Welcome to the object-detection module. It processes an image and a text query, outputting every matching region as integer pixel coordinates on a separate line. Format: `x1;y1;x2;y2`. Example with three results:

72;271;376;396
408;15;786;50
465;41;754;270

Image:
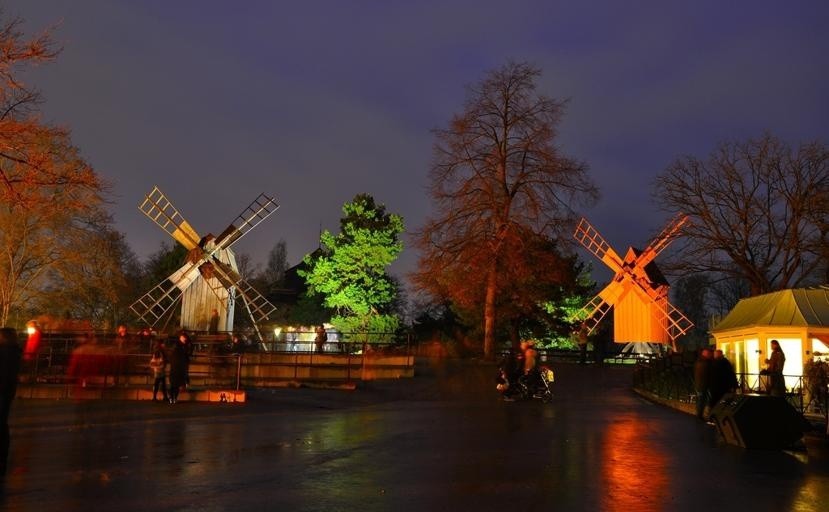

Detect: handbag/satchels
497;375;509;392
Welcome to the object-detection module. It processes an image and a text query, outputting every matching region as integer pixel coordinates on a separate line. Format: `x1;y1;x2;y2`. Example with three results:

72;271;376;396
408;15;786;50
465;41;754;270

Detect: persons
764;339;787;398
208;309;220;334
714;349;739;399
169;330;191;404
494;348;516;402
314;323;329;352
147;339;169;402
519;340;541;399
21;317;151;404
696;349;713;421
0;325;18;481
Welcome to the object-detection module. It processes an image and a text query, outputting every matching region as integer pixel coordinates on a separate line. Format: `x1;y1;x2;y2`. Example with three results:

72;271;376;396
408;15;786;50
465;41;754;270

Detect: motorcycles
492;357;557;406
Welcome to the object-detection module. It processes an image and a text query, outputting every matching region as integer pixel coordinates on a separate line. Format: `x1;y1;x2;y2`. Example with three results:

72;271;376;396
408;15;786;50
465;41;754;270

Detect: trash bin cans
708;393;816;449
